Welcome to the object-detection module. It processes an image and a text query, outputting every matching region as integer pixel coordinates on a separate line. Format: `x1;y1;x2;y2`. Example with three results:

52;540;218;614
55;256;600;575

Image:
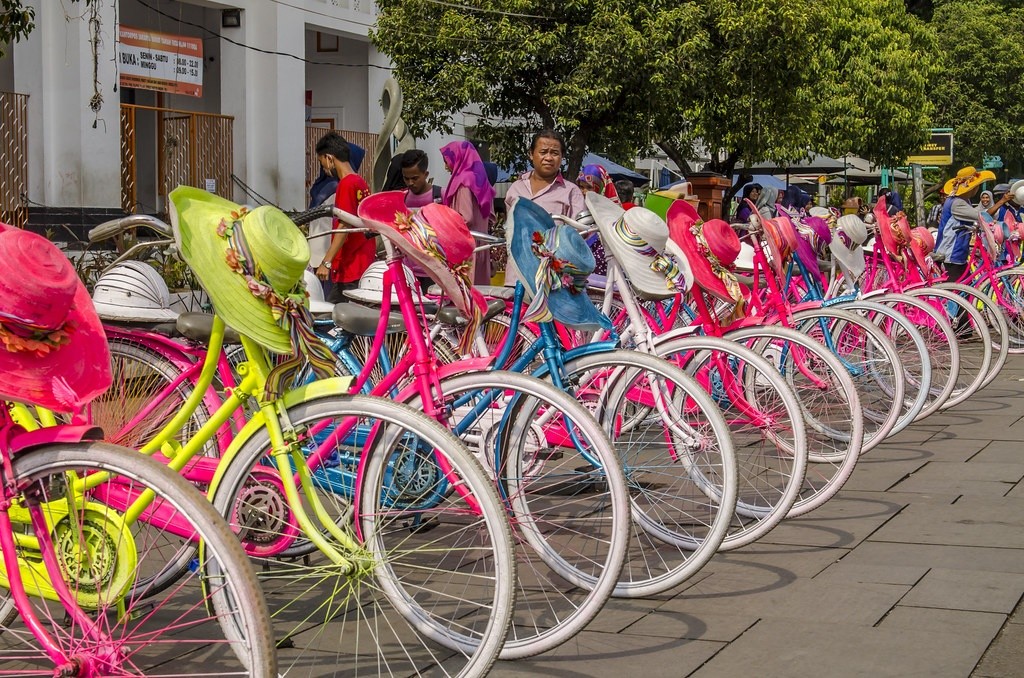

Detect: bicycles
0;191;1023;677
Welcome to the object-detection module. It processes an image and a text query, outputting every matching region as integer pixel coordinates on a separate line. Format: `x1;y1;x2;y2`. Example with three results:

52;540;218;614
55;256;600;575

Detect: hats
0;223;114;413
586;190;694;298
1009;179;1024;206
908;227;934;276
746;199;798;286
808;206;867;277
168;184;316;355
665;199;752;302
873;195;912;262
357;190;488;320
774;203;831;280
504;196;611;330
944;166;996;196
1002;209;1024;268
979;211;1003;263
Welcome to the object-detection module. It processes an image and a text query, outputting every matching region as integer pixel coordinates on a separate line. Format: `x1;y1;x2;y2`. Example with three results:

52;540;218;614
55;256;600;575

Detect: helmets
304;269;335;313
91;258;180;322
342;262;438;304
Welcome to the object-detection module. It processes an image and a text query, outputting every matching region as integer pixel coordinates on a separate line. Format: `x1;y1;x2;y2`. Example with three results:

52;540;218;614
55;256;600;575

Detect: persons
926;166;1024;342
307;128;640;297
735;184;903;276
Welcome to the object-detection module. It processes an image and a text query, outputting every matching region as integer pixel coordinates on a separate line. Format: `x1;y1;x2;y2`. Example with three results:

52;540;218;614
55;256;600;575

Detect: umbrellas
490;150;651;188
658;148;937;197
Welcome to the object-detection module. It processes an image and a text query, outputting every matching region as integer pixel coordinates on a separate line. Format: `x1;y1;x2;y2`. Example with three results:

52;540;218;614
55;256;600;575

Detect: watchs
322;261;332;269
